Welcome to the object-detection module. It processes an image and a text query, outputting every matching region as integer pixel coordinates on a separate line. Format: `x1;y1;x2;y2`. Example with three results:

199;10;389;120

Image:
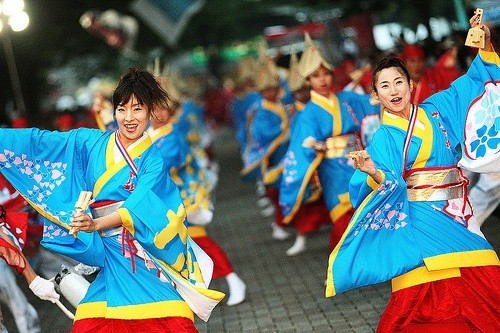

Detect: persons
0;5;500;333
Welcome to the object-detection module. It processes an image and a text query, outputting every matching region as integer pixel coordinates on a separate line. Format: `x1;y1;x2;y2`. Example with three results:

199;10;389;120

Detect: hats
154;58;184;108
288;48;310;92
299;30;336;77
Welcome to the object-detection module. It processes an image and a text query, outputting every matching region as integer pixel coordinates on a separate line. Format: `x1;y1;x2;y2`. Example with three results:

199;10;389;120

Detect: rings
356;163;361;166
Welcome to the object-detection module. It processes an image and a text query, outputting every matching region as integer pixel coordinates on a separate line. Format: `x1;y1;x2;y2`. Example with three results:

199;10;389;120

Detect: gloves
28;275;61;304
